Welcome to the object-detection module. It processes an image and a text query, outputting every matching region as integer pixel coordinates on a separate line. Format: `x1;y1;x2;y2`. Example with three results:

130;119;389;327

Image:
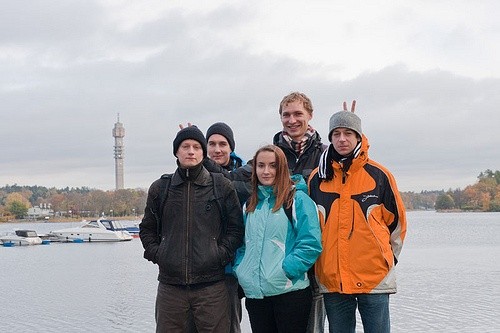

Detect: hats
328;112;363;142
206;122;235;152
173;125;207;159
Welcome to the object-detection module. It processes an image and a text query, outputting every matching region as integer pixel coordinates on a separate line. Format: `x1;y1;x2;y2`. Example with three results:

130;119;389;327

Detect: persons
206;122;246;333
139;127;246;333
308;111;407;333
224;144;323;333
178;92;356;333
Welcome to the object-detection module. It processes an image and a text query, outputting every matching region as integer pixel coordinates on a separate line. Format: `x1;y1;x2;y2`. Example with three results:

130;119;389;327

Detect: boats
0;230;49;246
50;216;132;242
124;227;140;238
